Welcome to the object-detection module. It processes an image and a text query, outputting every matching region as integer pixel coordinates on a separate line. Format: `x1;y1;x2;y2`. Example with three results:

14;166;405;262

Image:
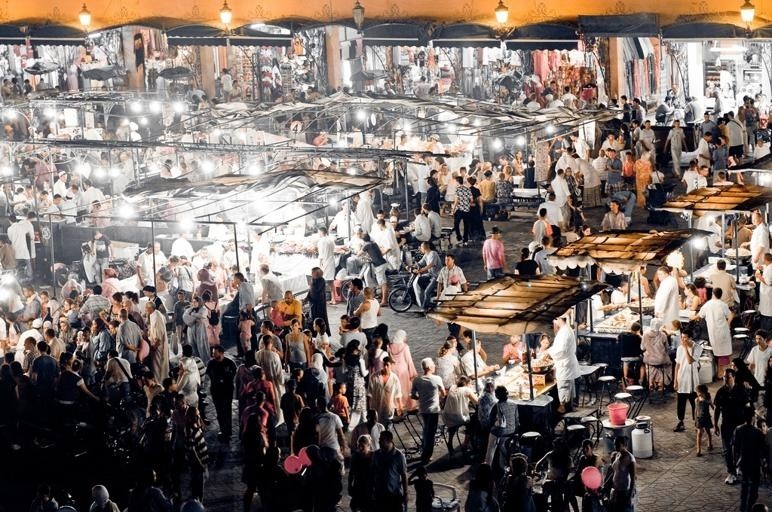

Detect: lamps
495;0;509;42
740;0;755;38
218;0;233;32
79;3;91;32
351;0;365;34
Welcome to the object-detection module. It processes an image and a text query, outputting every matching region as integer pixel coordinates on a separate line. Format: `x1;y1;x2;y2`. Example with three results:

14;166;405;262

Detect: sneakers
673;421;743;485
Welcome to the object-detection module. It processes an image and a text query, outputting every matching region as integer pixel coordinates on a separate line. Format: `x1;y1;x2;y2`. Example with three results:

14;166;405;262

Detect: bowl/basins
519;362;555;373
724;253;752;261
626;302;654;314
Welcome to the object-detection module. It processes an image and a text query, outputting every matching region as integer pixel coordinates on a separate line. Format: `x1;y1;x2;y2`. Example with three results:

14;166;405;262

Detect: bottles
756;269;761;283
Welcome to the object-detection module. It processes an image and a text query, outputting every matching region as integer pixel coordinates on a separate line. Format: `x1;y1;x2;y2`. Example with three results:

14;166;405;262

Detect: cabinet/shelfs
702;59;736;99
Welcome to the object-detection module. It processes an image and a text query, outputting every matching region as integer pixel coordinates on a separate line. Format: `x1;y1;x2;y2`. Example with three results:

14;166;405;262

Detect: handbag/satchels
129;378;141;394
493;411;508;429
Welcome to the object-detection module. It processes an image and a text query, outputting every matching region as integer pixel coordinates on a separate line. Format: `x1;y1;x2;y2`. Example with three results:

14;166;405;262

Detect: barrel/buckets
631;422;654;459
606;402;630;425
699;355;714;384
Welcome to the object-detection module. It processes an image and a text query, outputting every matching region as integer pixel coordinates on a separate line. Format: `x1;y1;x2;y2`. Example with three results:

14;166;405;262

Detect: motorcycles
389;263;444;314
340;251;398;305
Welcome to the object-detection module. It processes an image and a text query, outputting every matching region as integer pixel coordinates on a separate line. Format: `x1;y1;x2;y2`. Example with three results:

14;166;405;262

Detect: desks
510;174;548;210
641;106;720;162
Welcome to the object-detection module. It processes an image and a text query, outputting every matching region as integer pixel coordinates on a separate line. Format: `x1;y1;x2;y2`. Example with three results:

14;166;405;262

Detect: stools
732;309;757;346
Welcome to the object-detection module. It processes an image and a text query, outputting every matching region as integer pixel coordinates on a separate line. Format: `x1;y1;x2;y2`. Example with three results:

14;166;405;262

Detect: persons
0;41;771;511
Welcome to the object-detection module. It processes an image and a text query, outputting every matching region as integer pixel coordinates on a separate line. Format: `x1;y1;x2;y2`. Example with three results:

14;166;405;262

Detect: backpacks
203;302;220;326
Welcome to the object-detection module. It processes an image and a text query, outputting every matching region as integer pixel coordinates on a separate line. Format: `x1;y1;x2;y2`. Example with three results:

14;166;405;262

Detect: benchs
418;232;448;243
441;226;456;248
